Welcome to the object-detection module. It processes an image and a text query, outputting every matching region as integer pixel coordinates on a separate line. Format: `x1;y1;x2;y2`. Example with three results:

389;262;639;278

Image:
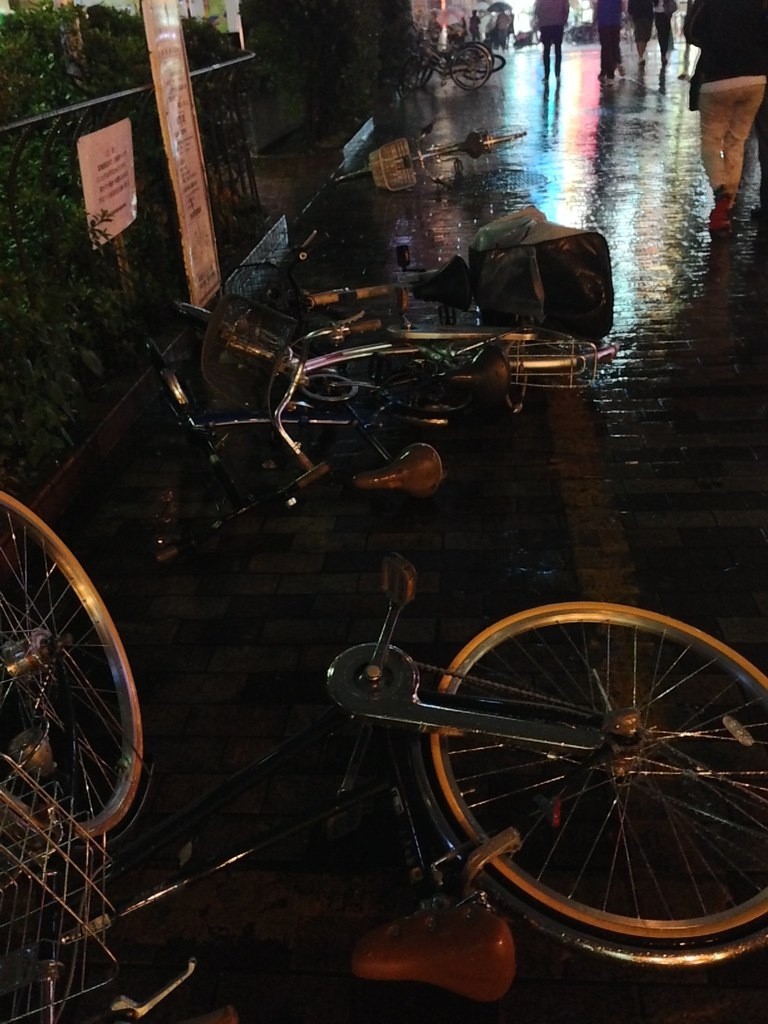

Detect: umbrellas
487;2;511;12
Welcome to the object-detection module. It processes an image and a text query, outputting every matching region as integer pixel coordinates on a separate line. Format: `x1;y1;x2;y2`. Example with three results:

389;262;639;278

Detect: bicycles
333;118;525;191
140;207;622;565
0;486;766;1024
393;14;507;98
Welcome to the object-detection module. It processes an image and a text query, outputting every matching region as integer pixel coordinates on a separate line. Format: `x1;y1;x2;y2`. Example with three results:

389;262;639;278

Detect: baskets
0;749;119;1024
200;292;299;417
223;262;288;312
369;138;417;192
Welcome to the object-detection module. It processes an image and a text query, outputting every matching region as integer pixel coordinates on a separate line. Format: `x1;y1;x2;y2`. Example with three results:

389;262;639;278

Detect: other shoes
607;79;617;85
598;75;606;84
618;64;625;76
751;206;768;219
661;56;668;65
678;74;686;79
638;57;646;66
710;193;731;229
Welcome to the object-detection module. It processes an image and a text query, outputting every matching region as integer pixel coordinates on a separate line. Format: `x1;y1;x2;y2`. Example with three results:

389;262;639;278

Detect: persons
208;16;220;33
417;9;511;53
535;0;570;80
683;0;768;232
591;0;677;86
